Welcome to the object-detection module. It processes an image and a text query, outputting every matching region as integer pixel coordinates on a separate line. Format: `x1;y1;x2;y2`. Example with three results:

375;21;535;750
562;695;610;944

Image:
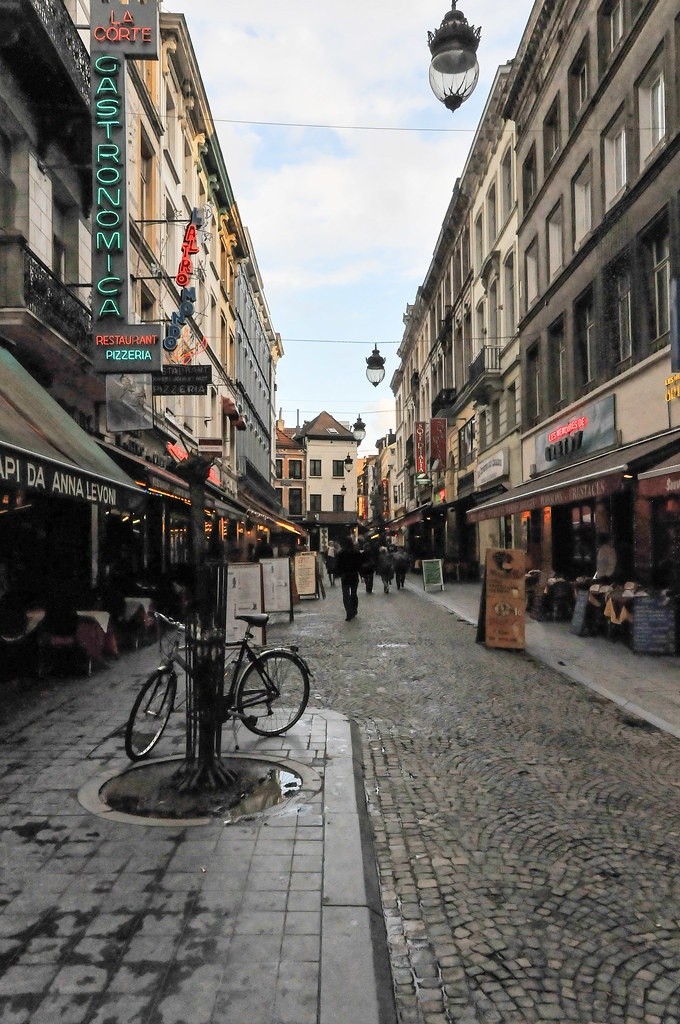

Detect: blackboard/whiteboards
151;364;213;395
422;558;445;592
632;596;678;658
569;590;591;636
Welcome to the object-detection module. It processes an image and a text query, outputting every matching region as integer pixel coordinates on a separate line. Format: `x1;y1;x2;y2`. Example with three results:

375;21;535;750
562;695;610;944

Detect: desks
0;580;165;687
524;569;680;647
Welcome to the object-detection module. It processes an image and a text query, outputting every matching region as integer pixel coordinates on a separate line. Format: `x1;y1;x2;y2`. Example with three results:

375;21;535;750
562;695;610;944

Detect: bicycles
123;610;316;761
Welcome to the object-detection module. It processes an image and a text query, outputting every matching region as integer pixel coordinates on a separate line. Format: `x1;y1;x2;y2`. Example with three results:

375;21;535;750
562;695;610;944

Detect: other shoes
346;608;359;620
383;587;390;593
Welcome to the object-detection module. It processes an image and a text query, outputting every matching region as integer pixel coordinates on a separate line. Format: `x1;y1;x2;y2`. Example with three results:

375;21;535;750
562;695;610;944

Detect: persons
357;547;369;583
252;534;274;566
392;546;411;590
377;544;394;594
268;536;338;587
592;530;620;582
333;536;366;624
246;541;256;563
359;541;378;595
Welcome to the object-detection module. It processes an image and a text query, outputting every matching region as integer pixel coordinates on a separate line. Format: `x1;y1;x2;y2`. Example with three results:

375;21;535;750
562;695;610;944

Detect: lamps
343;452;353;473
426;0;483;113
340;483;347;497
365;341;388;389
352;413;367;446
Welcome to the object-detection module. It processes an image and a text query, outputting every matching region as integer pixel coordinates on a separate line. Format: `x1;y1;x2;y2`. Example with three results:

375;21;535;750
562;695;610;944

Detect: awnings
86;431;214;512
242;506;303;538
465;433;680;523
0;328;145;516
636;444;680;500
189;480;249;525
